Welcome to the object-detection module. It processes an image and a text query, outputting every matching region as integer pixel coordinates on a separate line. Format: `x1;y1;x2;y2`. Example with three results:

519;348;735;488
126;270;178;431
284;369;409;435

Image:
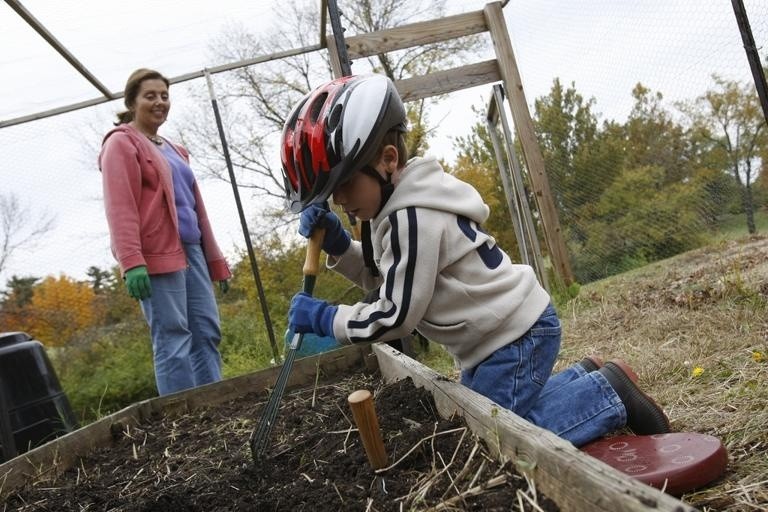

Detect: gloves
287;291;339;342
297;206;350;255
219;277;230;293
124;265;153;301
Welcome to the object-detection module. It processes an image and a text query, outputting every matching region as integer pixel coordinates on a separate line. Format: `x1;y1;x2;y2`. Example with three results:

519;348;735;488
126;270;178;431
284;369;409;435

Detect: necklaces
146;137;163;145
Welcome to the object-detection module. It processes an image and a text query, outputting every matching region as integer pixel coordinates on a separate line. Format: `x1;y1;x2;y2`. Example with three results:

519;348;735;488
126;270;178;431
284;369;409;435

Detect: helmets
279;72;407;215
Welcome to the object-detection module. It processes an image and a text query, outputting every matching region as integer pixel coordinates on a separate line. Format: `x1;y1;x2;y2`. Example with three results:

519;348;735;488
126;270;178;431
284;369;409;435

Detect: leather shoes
581;355;672;437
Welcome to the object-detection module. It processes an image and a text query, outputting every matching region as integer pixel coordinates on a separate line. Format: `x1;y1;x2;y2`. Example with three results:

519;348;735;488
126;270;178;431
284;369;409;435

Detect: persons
98;68;232;397
277;74;670;448
